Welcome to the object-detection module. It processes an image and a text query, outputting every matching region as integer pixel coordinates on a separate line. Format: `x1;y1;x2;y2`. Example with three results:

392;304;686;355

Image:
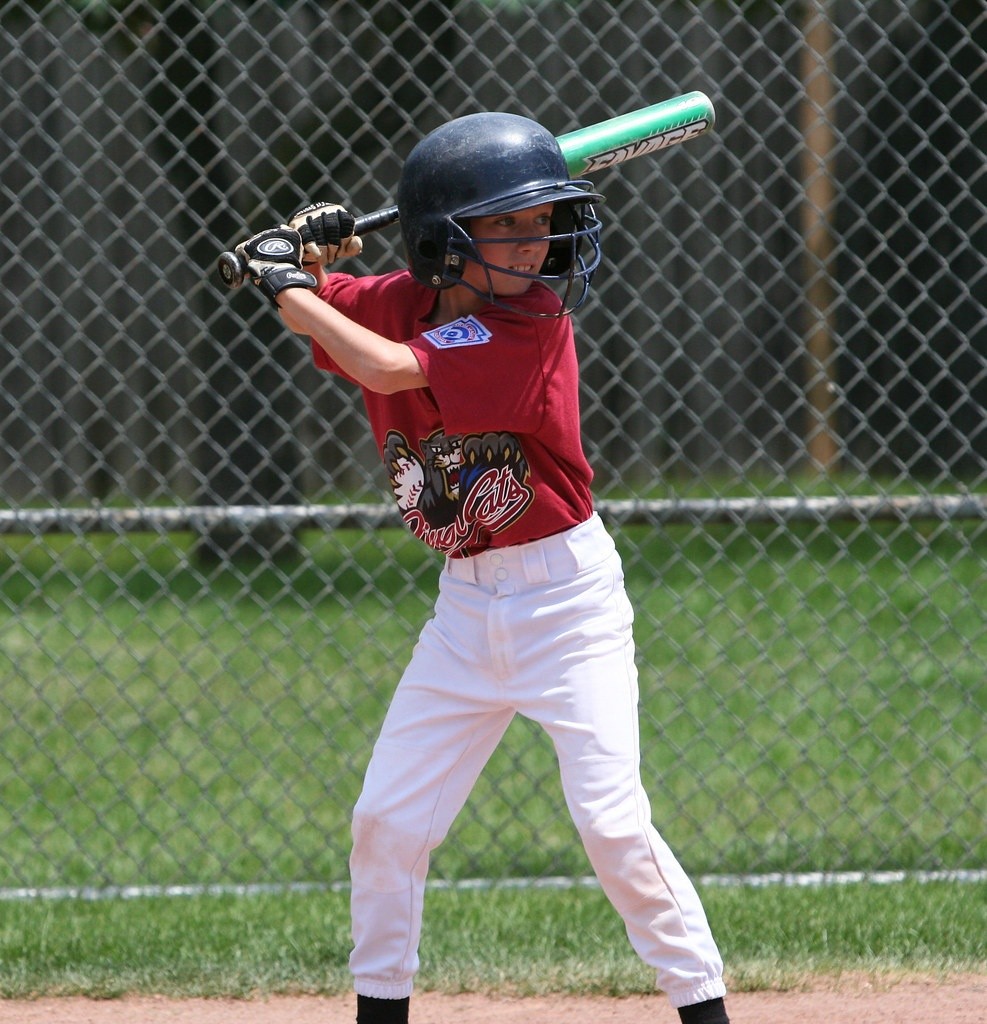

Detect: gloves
235;224;317;312
287;201;363;267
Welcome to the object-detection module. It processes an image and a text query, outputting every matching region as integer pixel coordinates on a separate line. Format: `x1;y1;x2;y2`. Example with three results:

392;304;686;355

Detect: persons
235;111;731;1024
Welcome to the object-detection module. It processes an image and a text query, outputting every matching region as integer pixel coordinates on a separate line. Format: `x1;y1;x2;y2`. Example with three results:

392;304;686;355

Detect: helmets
397;112;606;320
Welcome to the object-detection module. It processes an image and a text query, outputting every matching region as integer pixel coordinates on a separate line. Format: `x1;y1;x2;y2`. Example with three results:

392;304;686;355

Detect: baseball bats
219;90;718;289
